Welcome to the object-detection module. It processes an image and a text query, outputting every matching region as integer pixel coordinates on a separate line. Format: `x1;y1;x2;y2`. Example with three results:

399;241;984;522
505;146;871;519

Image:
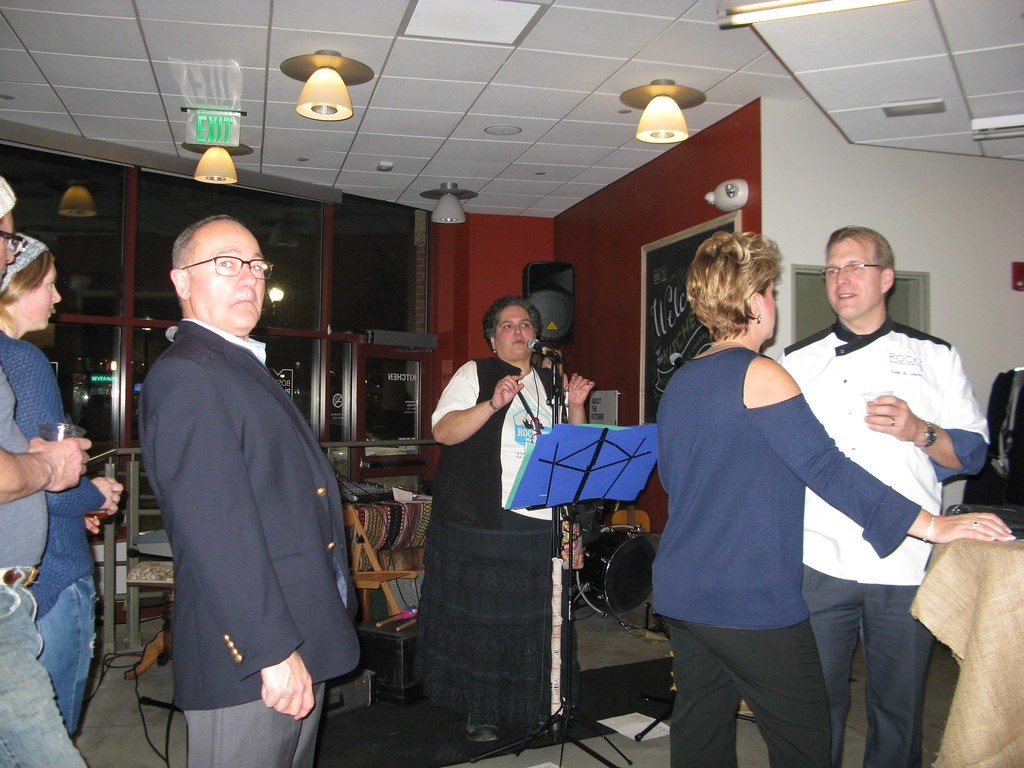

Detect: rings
890;417;895;427
499;379;503;383
972;521;980;529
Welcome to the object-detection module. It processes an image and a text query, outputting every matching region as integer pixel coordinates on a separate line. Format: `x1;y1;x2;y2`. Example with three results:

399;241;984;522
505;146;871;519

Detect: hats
0;175;17;220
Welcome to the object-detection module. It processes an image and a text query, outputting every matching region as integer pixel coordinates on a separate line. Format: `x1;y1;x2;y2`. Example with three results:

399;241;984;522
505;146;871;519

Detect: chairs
344;509;417;622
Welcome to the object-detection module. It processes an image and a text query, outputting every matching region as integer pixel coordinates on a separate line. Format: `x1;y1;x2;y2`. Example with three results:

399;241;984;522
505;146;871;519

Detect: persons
651;234;1016;768
0;173;123;768
418;298;595;742
138;214;362;768
770;225;991;768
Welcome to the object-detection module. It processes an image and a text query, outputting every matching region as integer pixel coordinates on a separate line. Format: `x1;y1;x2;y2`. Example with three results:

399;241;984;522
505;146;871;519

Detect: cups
38;422;86;442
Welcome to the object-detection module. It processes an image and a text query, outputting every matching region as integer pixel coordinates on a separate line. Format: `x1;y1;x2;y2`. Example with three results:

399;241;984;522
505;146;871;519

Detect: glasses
820;264;879;280
0;229;26;255
183;255;275;280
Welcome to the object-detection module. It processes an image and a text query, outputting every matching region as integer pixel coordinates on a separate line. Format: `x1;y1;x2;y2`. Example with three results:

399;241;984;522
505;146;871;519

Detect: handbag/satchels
560;506;584;569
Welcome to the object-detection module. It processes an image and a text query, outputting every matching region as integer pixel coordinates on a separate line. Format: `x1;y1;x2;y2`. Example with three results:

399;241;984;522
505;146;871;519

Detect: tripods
468;364;634;768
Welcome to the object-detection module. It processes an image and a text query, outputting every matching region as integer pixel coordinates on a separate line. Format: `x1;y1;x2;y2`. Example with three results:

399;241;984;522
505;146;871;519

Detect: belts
1;567;39;587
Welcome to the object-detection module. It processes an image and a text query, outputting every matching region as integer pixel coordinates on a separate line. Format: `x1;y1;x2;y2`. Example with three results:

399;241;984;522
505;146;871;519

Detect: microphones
527;338;563;364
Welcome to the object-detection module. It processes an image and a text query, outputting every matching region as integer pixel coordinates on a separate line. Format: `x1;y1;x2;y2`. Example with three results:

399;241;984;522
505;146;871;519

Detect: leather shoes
466;709;549;741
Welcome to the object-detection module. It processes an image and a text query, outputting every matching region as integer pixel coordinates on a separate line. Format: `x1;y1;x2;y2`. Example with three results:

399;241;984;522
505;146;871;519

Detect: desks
342;491;442;621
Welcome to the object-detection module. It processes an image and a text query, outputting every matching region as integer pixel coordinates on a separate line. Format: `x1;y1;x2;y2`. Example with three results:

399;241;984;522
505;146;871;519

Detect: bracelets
489;400;499;412
922;515;935;542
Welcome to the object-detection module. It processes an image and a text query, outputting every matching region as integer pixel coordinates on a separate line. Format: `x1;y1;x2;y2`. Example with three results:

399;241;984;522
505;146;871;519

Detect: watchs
914;418;937;448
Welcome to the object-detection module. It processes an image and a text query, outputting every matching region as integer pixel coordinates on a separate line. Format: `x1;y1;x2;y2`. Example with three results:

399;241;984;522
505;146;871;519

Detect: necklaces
710;341;754;351
529;364;543;419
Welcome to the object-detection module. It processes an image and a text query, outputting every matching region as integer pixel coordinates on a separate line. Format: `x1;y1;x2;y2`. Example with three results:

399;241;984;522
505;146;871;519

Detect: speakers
522;260;576;342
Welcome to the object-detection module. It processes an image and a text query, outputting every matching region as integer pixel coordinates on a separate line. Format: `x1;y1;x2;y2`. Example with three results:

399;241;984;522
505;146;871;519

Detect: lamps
419;183;479;223
621;77;707;145
716;0;913;31
275;51;377;122
179;141;251;187
970;112;1024;139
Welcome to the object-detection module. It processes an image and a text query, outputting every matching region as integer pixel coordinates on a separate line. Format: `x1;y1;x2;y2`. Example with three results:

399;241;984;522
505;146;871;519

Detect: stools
123;560;186;681
